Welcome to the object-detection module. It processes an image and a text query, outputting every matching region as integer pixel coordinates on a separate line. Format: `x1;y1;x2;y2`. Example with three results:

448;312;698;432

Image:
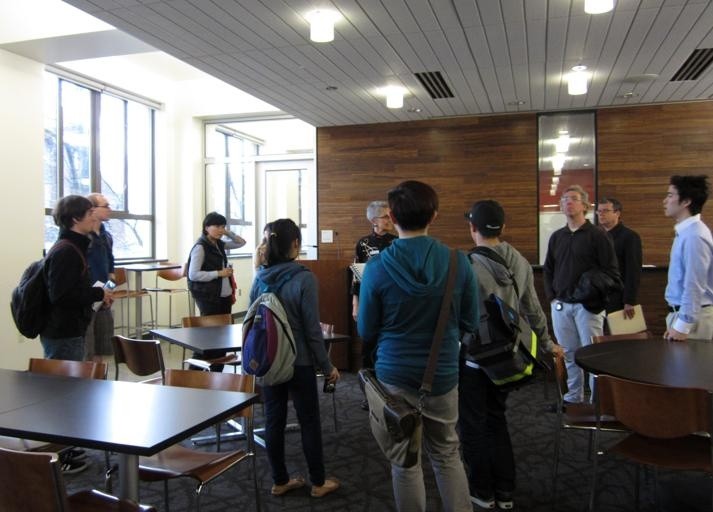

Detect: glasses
595;208;614;215
96;204;109;207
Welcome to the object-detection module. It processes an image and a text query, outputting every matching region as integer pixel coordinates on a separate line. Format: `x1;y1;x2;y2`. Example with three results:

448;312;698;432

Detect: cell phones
104;280;116;291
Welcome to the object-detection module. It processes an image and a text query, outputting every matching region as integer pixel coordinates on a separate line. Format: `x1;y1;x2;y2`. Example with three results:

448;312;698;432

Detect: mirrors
536;109;597;266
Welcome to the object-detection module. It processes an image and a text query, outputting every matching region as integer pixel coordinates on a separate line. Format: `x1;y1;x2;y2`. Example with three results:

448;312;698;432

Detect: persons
185;211;247;372
349;199;400;411
541;183;621;415
457;196;566;510
355;177;483;512
247;217;343;498
595;195;643;335
661;172;713;341
84;192;115;360
39;194;115;361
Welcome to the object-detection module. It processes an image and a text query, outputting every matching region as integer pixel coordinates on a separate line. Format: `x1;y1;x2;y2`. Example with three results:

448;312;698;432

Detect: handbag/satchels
467;292;552;392
357;368;422;468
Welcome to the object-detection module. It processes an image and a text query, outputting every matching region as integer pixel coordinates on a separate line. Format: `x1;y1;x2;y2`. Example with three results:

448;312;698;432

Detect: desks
150;322;346;447
114;263;181;334
0;366;256;507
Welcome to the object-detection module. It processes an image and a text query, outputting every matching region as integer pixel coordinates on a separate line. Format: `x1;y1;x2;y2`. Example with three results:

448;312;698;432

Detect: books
605;304;647;337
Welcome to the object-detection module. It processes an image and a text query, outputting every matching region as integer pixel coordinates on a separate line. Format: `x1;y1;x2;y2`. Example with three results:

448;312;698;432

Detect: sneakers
60;448;88;474
470;489;514;509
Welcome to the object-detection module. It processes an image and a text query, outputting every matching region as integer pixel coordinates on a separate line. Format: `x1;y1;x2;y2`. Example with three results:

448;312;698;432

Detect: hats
464;200;505;229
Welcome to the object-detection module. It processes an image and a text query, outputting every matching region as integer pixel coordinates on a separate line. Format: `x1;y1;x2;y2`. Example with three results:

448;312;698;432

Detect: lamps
584;0;613;14
568;78;587;95
549;131;568;196
386;90;403;109
309;15;334;42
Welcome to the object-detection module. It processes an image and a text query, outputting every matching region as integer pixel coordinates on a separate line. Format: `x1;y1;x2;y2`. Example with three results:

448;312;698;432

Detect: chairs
0;449;154;509
106;372;256;508
25;358;108;382
181;313;241;375
553;331;712;512
110;265;155;336
0;261;352;512
142;262;193;328
109;335;170;388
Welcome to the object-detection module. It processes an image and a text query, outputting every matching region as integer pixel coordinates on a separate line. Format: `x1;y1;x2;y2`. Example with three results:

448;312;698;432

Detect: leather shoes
271;478;304;495
311;478;340;497
545;400;582;413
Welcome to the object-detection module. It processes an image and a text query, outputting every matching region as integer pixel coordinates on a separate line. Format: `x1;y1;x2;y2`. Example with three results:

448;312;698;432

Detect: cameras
323;378;336;393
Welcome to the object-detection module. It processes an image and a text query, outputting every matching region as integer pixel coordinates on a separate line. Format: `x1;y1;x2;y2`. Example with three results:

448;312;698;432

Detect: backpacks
241;267;310;387
10;240;87;339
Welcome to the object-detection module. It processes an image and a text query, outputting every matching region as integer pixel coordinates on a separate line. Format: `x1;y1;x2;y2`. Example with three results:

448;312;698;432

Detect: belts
667;304;709;312
